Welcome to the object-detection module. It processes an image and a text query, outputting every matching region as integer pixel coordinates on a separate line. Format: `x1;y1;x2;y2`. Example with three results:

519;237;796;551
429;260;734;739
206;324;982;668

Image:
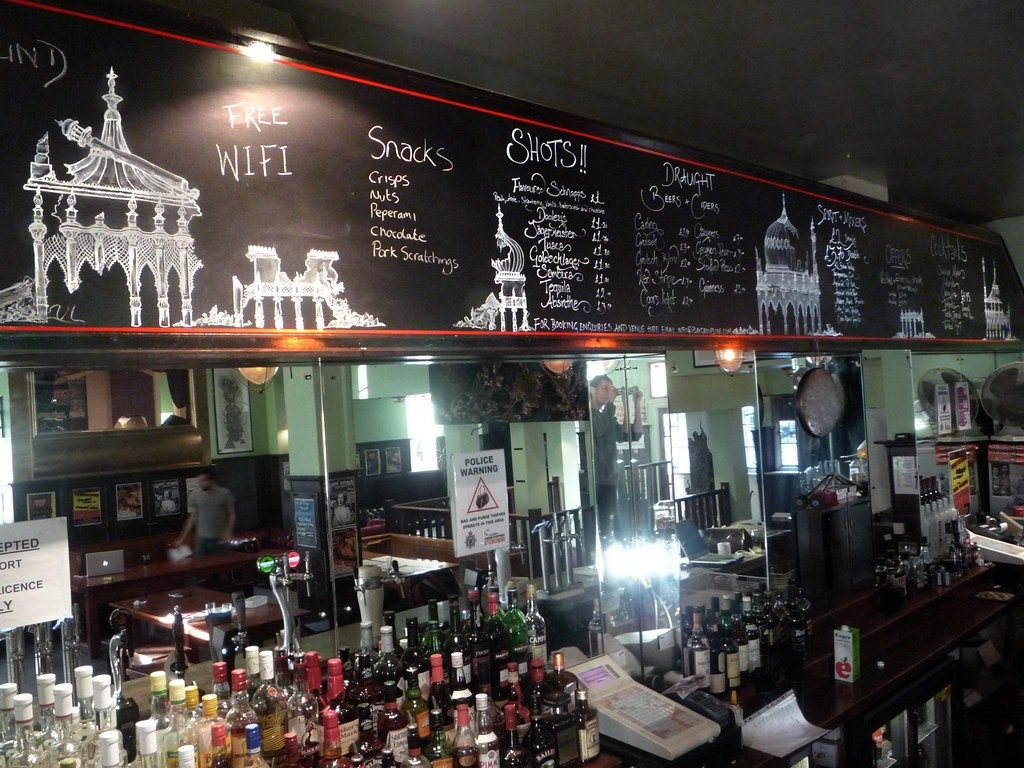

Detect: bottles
875;475;982;599
849;459;861;481
0;520;601;768
683;578;813;694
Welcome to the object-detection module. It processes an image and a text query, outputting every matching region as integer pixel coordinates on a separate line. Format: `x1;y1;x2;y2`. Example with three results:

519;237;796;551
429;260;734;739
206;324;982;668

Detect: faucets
282;553;292;603
303;550;314;597
391;560;405;598
120;614;135;662
231;591;247;659
352;566;367;607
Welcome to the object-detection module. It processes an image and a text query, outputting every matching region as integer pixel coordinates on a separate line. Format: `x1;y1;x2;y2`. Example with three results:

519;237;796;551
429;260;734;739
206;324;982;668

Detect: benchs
70;526;292;581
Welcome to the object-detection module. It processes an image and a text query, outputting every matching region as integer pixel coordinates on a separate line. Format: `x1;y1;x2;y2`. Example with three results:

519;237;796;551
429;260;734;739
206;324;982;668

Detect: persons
589;375;643;540
172;469;236;556
160;489;175;513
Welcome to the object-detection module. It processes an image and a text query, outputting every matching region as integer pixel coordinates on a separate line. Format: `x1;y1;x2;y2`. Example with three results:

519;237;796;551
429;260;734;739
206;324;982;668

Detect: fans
917;367;988;435
980;361;1024;432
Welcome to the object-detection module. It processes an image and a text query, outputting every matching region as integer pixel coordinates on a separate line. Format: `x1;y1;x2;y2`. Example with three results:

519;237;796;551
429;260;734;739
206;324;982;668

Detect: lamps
714;349;745;373
237;367;278;393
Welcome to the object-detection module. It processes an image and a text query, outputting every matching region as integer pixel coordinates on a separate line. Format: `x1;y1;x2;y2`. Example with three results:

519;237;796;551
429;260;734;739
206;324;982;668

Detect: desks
111;582;313;656
361;553;459;610
72;549;289;659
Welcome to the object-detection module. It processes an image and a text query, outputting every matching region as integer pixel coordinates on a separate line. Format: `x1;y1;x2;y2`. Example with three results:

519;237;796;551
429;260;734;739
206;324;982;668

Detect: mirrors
0;351;1024;701
31;369;197;444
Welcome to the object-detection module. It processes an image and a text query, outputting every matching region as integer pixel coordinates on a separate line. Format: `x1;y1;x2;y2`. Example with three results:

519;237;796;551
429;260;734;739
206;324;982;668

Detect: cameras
628;387;635;395
616;387;625;394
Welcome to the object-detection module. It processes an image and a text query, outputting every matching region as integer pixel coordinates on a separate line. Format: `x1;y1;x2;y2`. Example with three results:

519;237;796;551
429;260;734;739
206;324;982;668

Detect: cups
717;542;731;556
